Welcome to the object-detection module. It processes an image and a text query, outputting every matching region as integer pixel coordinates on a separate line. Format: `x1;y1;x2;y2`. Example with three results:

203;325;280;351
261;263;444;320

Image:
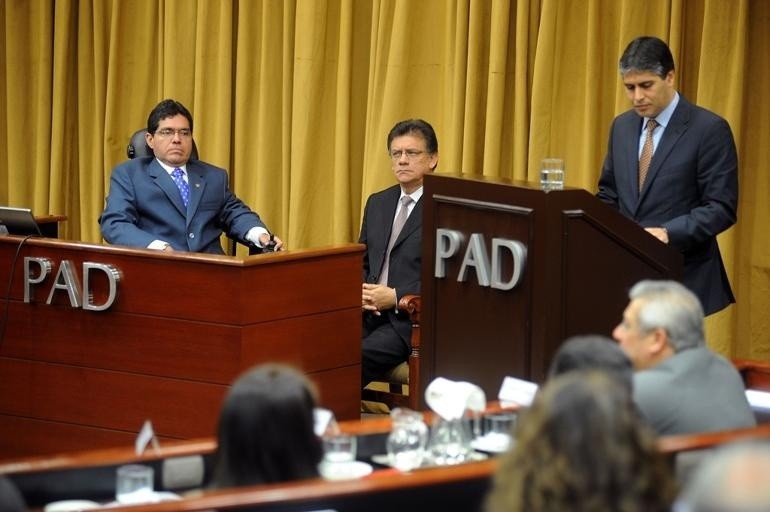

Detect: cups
485;411;517;451
541;158;565;190
386;407;427;469
323;434;356;465
116;465;153;505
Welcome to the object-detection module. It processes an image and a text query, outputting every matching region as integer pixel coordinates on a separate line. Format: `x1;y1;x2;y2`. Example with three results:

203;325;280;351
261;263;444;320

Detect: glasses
152;129;191;138
389;150;430;160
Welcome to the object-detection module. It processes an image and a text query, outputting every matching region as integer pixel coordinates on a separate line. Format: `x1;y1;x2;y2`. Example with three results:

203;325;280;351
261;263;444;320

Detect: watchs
162;243;171;251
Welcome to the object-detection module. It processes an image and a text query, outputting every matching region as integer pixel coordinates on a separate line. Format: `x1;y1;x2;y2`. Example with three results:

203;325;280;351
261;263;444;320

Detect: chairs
128;127;283;255
362;295;421;410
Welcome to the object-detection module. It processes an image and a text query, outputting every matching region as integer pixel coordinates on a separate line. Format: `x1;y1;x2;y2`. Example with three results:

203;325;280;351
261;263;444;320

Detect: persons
484;369;679;511
548;335;635;398
97;99;285;256
594;35;739;319
612;278;759;481
211;362;325;489
358;118;440;392
672;439;770;512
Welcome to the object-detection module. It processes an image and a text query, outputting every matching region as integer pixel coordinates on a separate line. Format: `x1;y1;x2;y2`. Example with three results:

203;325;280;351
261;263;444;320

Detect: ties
639;120;658;193
171;166;189;208
378;196;413;287
